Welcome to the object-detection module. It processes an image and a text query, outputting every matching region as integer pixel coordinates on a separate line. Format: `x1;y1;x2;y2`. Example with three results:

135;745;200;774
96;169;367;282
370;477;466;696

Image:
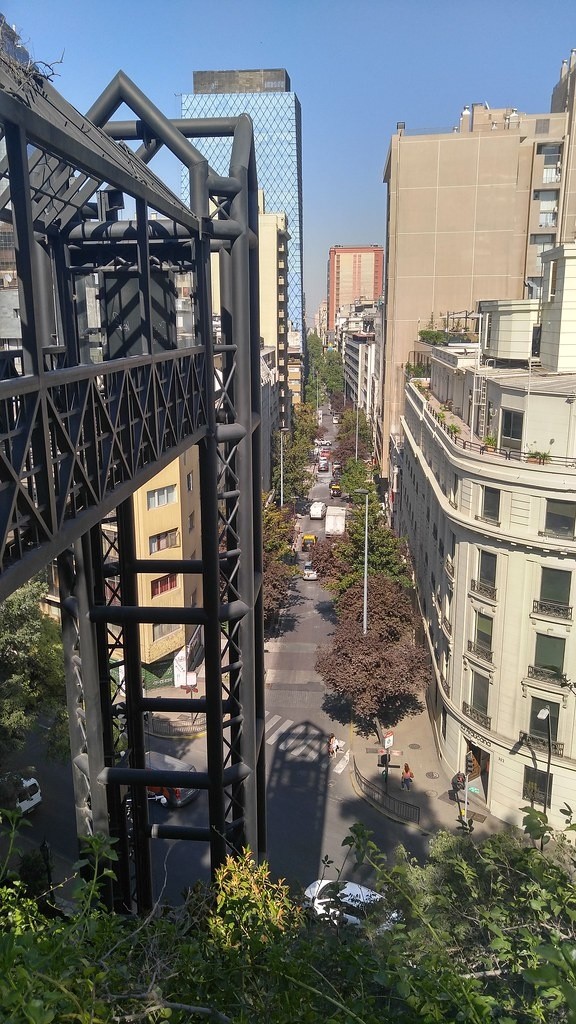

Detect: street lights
279;428;291;506
354;489;369;635
536;705;551;854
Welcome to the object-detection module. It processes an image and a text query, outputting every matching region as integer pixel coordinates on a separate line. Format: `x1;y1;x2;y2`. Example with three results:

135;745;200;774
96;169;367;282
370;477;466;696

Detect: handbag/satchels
409;769;414;778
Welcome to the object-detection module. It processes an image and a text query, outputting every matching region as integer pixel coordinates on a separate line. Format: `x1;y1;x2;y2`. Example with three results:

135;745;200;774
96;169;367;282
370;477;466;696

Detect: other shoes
406;790;410;792
400;788;404;791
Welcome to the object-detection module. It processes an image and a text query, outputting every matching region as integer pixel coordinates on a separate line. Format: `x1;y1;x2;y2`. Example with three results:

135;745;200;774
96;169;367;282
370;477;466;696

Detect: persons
401;763;414;791
328;733;338;758
452;773;466;802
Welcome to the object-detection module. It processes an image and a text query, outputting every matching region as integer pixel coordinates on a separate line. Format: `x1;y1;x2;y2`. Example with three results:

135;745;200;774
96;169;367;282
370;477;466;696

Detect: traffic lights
457;773;464;790
465;751;475;776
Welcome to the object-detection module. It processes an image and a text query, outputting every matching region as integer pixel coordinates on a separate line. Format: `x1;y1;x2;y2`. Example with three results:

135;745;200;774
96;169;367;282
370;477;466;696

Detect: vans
140;751;200;807
310;502;328;519
16;778;42;818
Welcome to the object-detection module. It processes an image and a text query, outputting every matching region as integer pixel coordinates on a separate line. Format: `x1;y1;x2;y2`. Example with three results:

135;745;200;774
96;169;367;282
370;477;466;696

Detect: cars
314;437;332;446
333;417;339;424
304;879;398;935
301;535;318;552
303;562;320;582
311;447;343;497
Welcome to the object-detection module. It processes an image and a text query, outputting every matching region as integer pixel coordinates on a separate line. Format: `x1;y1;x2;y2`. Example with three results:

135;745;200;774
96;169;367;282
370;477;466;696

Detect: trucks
324;506;349;538
